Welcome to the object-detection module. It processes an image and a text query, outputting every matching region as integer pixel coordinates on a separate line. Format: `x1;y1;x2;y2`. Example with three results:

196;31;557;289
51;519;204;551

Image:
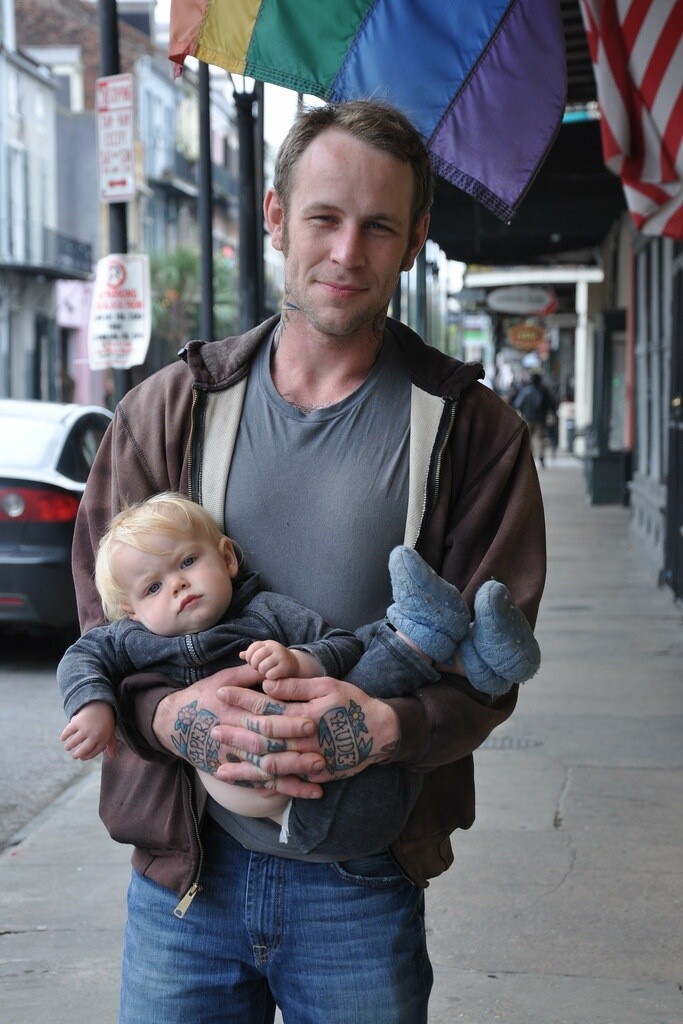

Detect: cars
0;395;116;628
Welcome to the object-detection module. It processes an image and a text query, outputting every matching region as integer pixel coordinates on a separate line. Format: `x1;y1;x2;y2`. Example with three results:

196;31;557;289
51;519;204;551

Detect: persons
56;494;540;858
512;373;557;467
71;101;546;1023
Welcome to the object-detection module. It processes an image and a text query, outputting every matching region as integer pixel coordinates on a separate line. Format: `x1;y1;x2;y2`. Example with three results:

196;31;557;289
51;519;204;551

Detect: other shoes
387;544;470;663
539;457;546;467
457;578;541;697
